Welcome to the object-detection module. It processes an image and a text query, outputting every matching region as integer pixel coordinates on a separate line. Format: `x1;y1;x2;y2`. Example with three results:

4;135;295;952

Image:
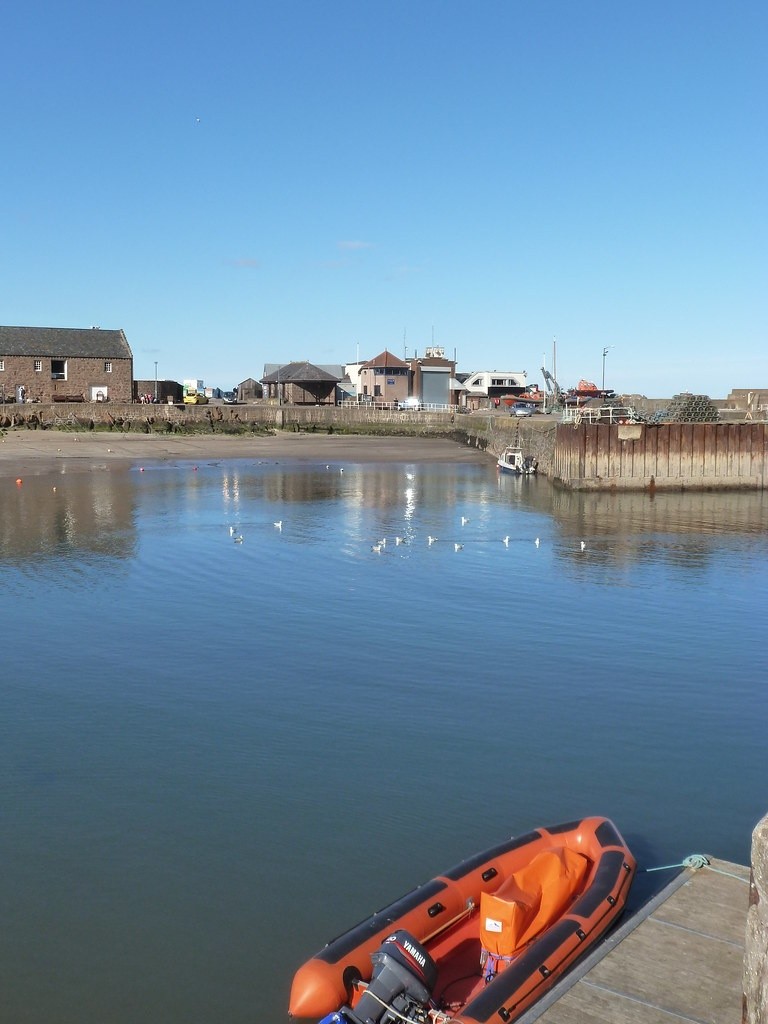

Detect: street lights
602;345;615;402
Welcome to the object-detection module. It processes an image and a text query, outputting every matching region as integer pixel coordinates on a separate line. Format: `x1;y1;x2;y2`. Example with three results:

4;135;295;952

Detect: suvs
398;396;423;411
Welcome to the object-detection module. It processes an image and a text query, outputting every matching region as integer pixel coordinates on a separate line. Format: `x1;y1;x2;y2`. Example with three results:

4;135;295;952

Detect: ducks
534;538;541;544
580;541;586;549
229;527;244;544
370;517;470;550
273;520;282;526
502;536;511;542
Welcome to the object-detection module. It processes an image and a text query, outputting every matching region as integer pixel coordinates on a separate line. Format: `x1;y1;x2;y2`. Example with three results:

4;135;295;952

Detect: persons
140;393;154;405
393;398;398;410
20;388;26;404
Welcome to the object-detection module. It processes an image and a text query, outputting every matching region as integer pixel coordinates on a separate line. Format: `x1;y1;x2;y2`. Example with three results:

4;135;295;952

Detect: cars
222;391;237;405
509;402;533;417
184;393;209;405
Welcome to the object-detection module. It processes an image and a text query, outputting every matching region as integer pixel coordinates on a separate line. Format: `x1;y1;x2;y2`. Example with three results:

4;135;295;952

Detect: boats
287;816;637;1024
496;445;536;473
